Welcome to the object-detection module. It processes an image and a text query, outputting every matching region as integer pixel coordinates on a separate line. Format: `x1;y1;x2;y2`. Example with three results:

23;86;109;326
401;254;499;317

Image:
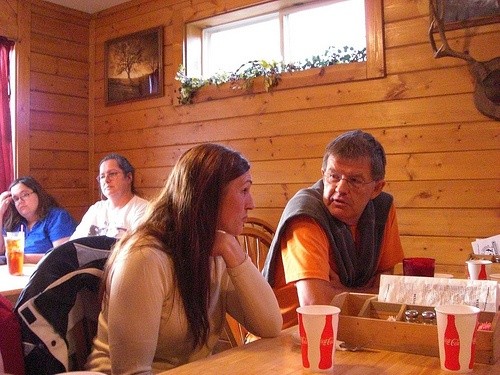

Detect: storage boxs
327;292;500;365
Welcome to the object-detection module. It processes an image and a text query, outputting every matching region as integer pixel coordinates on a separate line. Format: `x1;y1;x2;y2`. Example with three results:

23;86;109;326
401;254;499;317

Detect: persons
0;176;78;264
70;154;155;241
245;128;405;345
82;142;284;375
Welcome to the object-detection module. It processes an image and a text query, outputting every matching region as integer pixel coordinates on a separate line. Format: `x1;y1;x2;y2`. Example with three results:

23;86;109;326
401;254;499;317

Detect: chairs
13;218;278;375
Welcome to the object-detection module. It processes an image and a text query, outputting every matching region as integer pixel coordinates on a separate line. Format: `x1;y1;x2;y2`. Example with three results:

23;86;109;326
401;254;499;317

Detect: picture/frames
103;25;165;107
429;0;500;33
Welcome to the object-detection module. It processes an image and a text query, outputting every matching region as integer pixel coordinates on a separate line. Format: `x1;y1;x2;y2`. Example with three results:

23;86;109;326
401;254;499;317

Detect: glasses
324;170;372;191
96;171;123;182
13;191;33;201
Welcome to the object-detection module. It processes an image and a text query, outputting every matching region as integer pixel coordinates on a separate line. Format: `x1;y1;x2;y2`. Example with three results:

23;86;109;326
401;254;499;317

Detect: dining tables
0;262;39;304
153;323;500;375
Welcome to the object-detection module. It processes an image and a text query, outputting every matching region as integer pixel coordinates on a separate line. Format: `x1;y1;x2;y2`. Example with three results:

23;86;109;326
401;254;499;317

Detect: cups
433;305;481;374
490;273;500;282
434;274;455;279
4;236;26;274
8;231;25;239
466;259;493;280
402;257;436;277
296;304;341;370
